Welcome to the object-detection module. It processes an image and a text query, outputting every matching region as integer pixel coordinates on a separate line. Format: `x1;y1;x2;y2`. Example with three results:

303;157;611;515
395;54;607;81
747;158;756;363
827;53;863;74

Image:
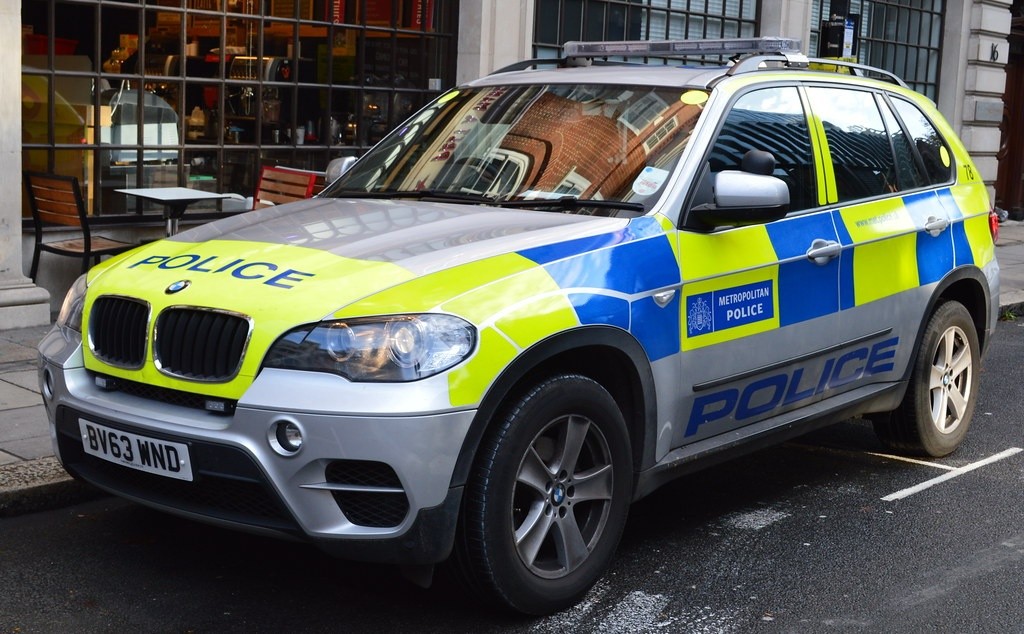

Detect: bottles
240;86;255;116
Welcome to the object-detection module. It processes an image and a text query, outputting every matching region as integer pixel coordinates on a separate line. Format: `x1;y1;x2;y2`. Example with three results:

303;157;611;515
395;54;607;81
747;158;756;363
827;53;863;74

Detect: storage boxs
155;0;435;33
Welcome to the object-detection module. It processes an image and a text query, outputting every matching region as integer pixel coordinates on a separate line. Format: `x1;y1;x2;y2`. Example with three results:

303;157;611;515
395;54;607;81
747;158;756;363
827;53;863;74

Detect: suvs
38;37;1001;618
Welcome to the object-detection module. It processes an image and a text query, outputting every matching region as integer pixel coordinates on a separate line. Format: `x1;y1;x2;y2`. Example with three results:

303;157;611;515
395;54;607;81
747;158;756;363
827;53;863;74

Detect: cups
271;129;281;145
296;127;304;144
232;131;244;144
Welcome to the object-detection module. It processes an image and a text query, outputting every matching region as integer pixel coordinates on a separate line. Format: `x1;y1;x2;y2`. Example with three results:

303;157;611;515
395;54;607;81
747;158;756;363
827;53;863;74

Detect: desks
112;187;232;238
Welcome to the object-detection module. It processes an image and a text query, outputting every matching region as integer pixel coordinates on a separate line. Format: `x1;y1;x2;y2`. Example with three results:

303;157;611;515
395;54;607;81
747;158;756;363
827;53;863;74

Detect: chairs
21;168;138;286
251;166;329;211
